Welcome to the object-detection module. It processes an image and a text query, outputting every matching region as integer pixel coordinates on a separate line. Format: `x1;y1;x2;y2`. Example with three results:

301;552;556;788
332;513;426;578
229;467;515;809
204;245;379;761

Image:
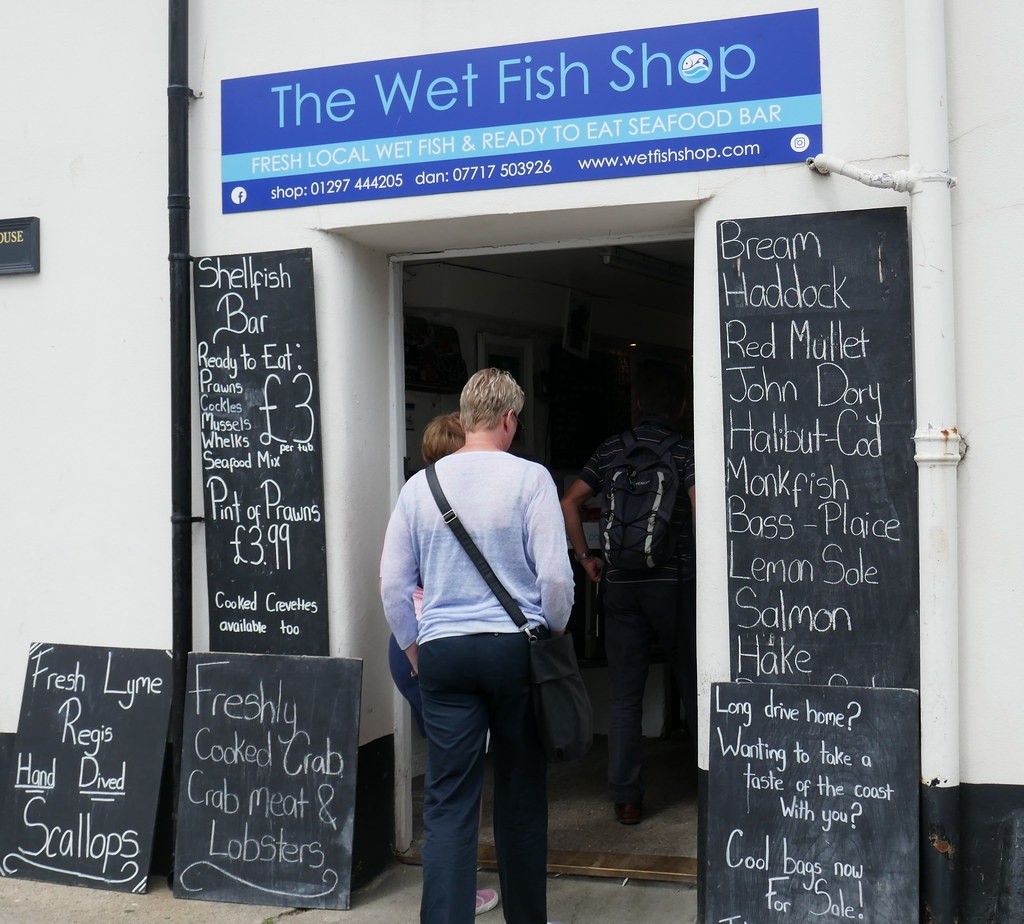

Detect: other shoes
475;889;498;916
615;803;640;825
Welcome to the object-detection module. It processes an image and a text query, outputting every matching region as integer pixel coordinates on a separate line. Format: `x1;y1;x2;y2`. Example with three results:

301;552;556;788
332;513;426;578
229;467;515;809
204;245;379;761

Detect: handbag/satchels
528;638;593;762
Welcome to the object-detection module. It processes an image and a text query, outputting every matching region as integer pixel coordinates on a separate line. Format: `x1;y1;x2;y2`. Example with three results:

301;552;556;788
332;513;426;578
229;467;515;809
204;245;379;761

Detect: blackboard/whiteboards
703;681;920;924
714;204;922;693
0;641;173;894
170;651;366;910
193;247;331;657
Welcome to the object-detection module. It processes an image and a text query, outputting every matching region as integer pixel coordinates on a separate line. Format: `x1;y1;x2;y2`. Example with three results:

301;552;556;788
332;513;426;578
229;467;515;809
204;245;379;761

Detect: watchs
574;550;593;563
408;668;418;683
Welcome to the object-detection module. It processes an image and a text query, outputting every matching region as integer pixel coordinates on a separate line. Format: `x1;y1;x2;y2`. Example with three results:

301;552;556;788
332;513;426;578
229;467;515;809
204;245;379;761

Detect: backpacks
600;429;685;570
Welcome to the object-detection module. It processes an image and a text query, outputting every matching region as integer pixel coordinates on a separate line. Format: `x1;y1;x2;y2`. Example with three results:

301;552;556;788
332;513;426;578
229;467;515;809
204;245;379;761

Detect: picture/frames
477;332;537;457
562;286;595;360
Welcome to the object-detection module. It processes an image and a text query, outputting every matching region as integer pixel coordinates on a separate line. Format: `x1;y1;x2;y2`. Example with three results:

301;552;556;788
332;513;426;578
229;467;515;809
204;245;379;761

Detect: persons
561;366;696;825
388;410;499;917
385;365;576;924
